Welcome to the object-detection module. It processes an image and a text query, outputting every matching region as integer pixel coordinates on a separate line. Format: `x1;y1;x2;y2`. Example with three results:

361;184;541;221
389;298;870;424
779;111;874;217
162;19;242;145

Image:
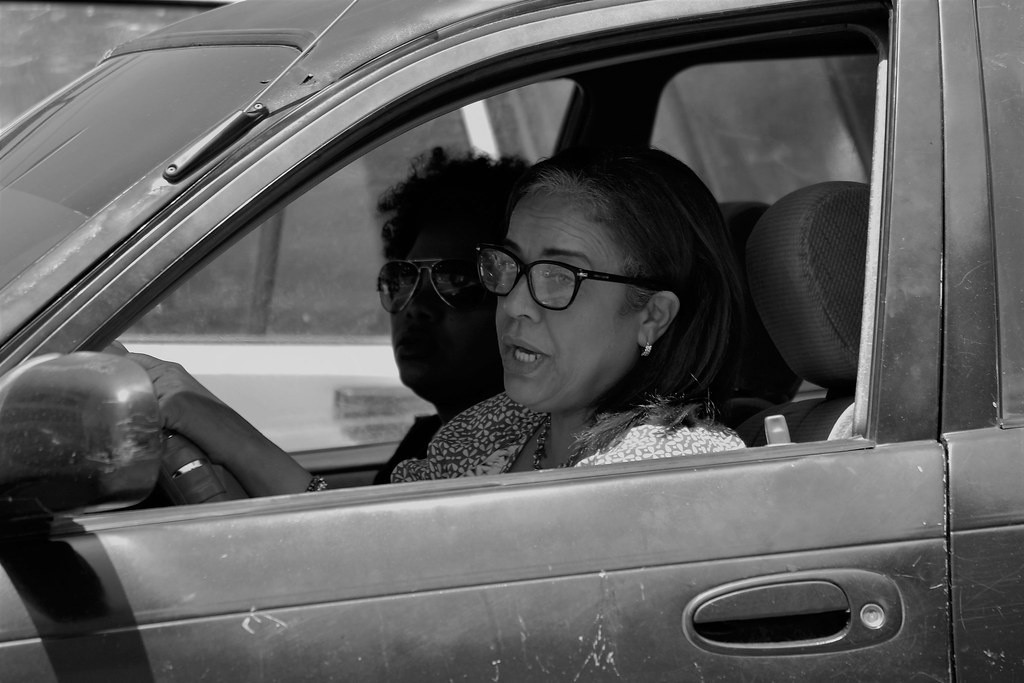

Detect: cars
0;0;1024;683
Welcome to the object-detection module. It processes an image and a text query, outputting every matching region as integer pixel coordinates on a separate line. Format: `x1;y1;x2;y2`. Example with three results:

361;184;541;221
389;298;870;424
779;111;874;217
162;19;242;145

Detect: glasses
377;257;489;315
474;242;679;312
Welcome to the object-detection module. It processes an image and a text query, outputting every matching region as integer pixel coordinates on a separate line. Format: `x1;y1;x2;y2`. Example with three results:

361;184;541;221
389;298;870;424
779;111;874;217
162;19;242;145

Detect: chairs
734;181;871;450
717;198;803;430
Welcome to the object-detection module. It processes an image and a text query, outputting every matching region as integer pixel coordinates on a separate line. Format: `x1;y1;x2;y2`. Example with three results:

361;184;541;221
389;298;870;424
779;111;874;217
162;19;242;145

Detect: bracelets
306;476;327;491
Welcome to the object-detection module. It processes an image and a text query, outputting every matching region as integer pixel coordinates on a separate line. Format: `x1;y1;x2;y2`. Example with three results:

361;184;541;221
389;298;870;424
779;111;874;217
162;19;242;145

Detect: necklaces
531;415;596;470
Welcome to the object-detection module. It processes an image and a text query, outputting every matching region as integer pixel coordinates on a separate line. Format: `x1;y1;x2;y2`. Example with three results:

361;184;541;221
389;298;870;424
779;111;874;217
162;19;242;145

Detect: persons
122;147;747;498
